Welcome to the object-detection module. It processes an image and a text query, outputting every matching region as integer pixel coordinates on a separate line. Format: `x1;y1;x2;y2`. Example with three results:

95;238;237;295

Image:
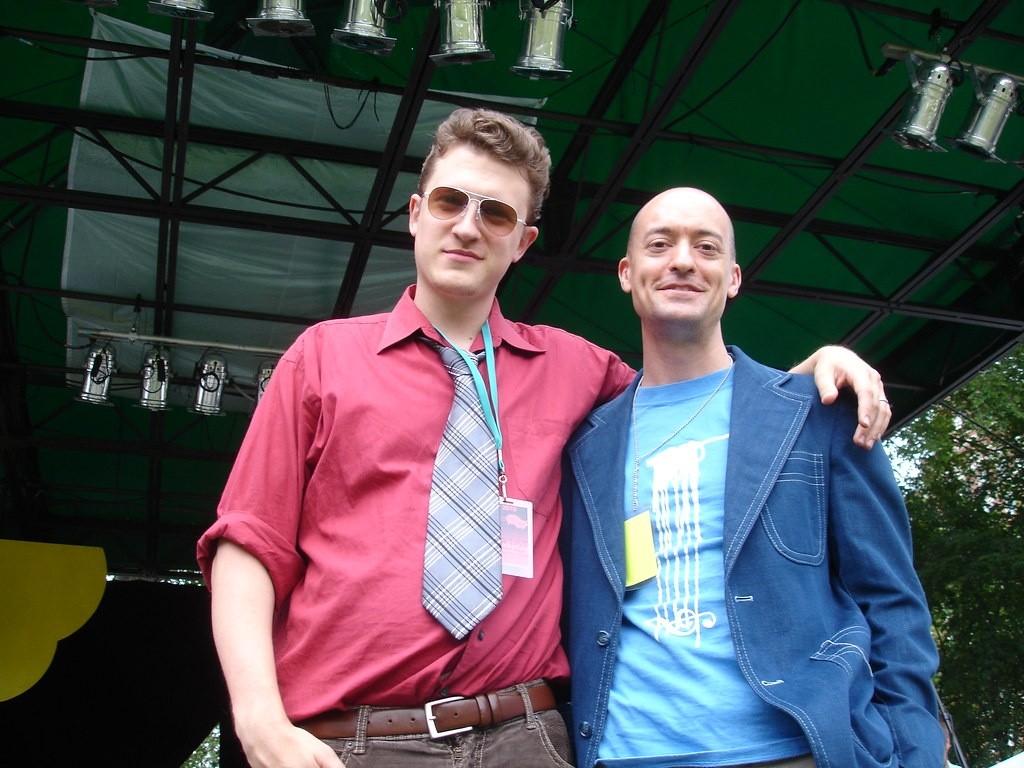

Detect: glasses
420;186;527;237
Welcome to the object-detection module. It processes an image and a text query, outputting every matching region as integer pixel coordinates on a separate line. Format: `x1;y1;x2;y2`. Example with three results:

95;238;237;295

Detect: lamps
245;0;316;37
186;347;236;416
252;354;284;406
428;0;496;69
132;343;180;411
883;51;964;153
73;338;121;407
941;64;1019;165
330;0;397;57
510;0;577;80
147;0;215;23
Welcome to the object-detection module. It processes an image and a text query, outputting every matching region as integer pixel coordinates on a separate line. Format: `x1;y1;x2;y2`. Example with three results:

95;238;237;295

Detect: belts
297;685;561;739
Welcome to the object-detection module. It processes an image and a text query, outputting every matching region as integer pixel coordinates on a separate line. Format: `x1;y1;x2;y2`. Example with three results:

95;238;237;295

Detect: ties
416;330;503;641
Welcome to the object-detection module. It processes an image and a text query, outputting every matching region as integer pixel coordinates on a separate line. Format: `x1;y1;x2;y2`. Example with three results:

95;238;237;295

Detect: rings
880;398;888;403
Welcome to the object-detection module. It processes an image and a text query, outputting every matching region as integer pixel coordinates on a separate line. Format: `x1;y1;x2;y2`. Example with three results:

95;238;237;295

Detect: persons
196;110;893;768
559;186;948;767
